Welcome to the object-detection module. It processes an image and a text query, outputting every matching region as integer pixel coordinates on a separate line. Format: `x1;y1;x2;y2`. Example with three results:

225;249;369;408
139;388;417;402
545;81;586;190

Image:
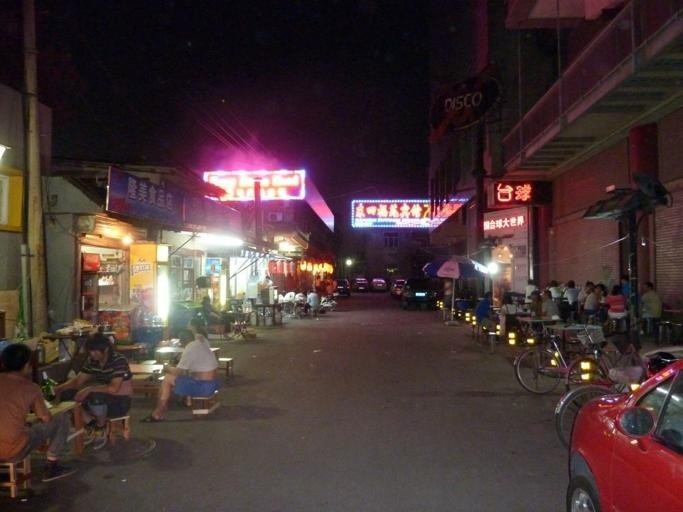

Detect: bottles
35;340;45;366
139;311;162;327
106;263;110;272
40;370;55;401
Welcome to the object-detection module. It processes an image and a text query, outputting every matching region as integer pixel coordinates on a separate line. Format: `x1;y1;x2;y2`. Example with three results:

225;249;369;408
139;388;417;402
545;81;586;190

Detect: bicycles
511;303;683;452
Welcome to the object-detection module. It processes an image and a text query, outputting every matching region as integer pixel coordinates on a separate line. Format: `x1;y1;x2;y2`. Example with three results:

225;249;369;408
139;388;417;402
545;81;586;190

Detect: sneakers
38;461;79;482
82;417;97;445
92;420;111;450
138;411;167;424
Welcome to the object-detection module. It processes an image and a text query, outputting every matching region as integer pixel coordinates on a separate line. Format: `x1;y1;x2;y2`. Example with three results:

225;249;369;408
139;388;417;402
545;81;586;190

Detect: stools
431;285;683;366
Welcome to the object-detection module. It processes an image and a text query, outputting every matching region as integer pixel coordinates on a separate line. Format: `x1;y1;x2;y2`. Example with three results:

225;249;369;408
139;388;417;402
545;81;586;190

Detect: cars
566;360;683;510
333;277;438;311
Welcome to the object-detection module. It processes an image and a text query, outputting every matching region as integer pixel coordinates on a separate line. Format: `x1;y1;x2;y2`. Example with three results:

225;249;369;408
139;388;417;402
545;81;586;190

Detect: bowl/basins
99;325;111;332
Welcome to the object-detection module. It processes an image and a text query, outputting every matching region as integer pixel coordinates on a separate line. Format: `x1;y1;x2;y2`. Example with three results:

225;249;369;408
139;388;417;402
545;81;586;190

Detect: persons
498;293;517;334
440;277;452;307
0;344;80;483
294;288;308;312
303;288;319;314
265;276;273;287
141;330;219;422
187;317;211;341
277;290;286;303
283;289;296;308
39;331;135;450
199;295;236;336
475;290;500;343
523;275;663;336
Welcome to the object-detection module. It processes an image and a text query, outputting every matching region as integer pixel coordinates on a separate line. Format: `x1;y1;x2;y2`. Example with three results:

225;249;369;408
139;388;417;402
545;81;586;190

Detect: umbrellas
423;253;490;321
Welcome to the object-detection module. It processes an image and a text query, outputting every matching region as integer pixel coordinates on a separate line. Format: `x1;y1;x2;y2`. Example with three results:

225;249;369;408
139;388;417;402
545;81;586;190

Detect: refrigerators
98;303;137;345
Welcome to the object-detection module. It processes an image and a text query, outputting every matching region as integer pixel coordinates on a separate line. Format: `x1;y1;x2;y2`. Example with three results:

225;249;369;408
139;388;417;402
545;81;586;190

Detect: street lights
344;259;352;280
